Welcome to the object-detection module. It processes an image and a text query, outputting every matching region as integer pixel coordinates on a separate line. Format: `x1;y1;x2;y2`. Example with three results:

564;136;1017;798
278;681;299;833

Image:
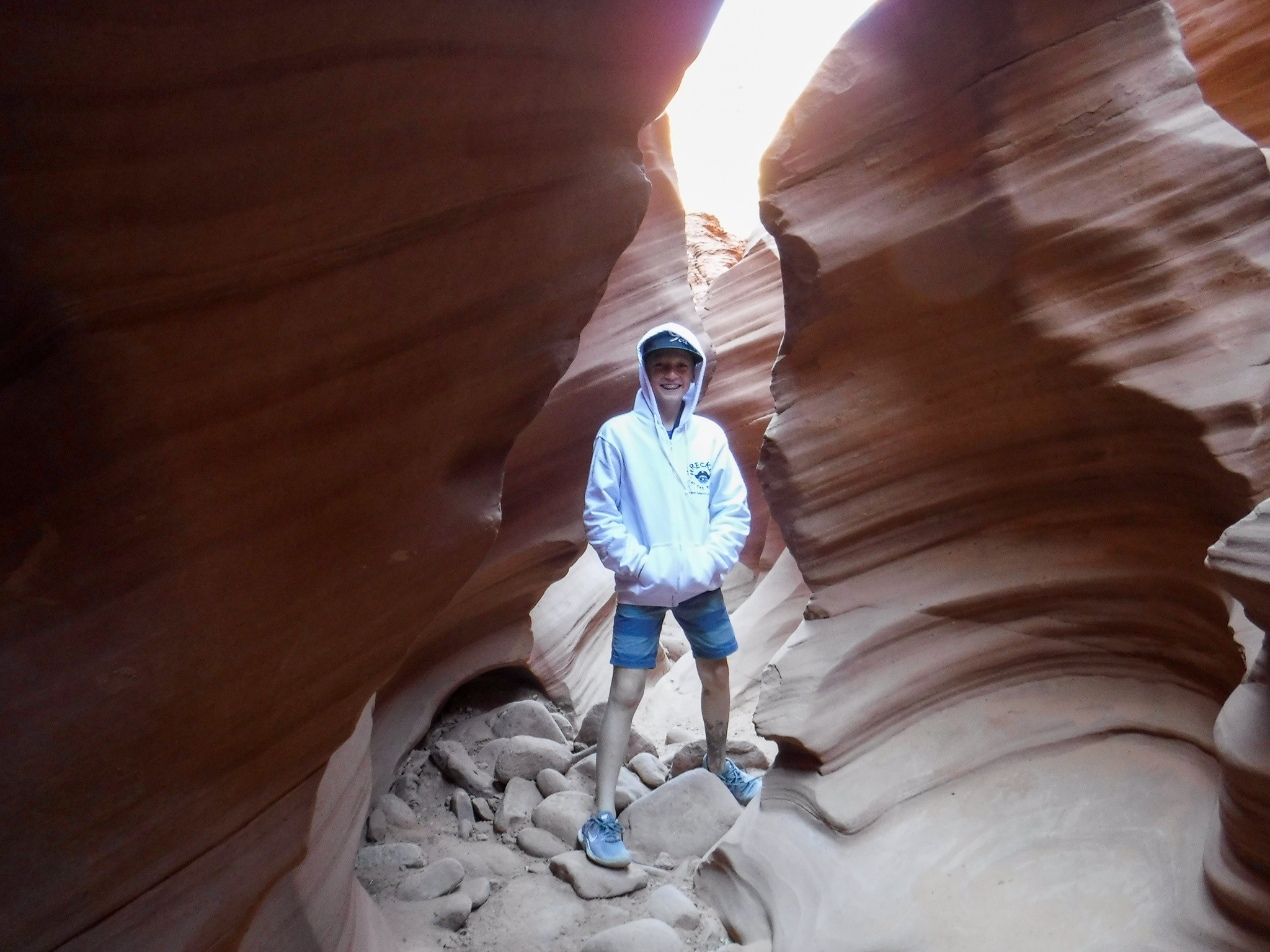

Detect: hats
642;330;703;365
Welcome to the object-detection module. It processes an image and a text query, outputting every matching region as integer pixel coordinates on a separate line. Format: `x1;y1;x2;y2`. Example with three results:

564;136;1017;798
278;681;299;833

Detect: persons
577;322;764;867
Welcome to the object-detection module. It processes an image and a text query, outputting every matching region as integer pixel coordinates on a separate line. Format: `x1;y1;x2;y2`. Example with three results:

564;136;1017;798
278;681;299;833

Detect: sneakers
702;753;762;806
577;810;631;868
